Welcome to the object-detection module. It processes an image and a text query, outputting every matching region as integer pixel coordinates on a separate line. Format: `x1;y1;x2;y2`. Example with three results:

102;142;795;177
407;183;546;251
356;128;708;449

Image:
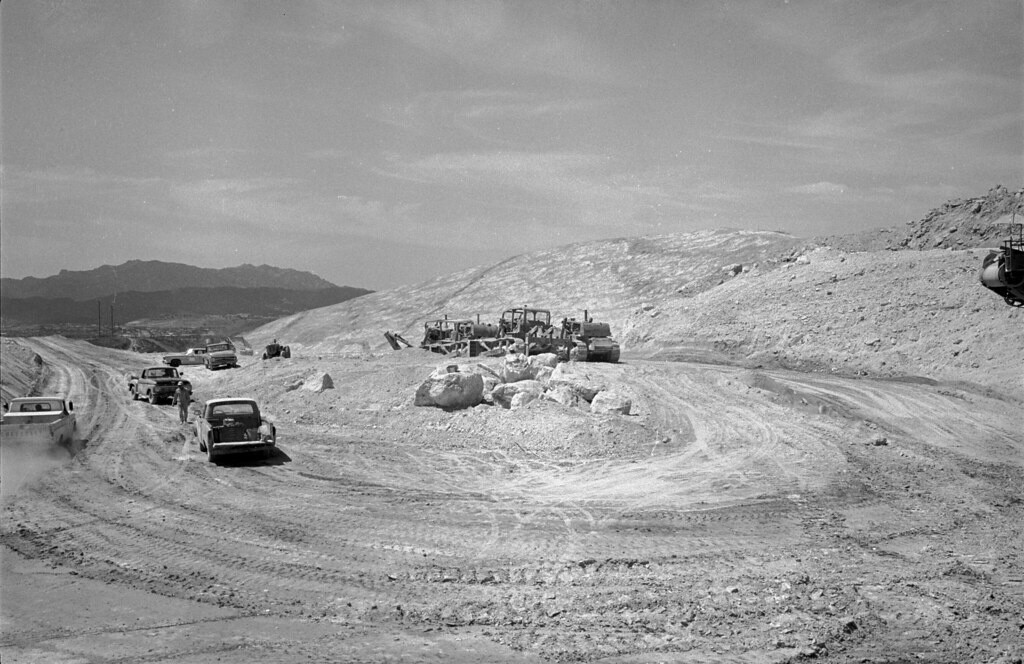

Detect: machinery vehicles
384;304;621;362
262;339;290;359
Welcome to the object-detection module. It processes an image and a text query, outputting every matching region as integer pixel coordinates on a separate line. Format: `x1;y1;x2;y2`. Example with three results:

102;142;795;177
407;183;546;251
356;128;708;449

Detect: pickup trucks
193;398;276;461
163;348;206;367
0;397;77;449
128;367;192;404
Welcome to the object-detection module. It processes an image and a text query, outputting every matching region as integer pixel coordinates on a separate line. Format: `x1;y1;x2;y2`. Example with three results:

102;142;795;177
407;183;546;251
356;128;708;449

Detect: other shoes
180;421;187;425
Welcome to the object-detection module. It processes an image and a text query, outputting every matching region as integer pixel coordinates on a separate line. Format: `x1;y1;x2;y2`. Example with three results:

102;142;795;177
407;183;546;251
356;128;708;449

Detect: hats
177;381;183;386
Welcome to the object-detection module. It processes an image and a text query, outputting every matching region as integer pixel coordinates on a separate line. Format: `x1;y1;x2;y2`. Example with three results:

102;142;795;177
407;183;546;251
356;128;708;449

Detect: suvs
203;342;238;370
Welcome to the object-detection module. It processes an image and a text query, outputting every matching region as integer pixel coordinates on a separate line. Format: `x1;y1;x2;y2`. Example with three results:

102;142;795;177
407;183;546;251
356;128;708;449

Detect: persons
36;404;43;411
171;381;190;425
